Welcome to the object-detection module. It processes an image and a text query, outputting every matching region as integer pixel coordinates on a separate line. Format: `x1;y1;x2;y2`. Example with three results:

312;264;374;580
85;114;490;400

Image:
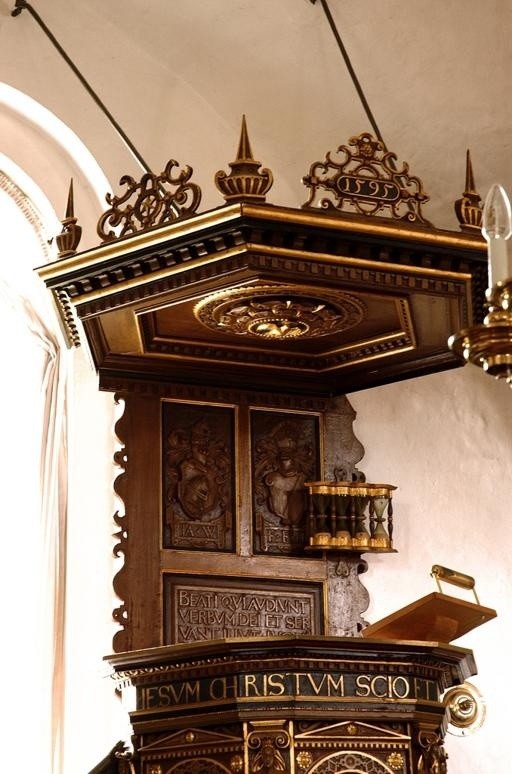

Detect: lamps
482;183;512;289
